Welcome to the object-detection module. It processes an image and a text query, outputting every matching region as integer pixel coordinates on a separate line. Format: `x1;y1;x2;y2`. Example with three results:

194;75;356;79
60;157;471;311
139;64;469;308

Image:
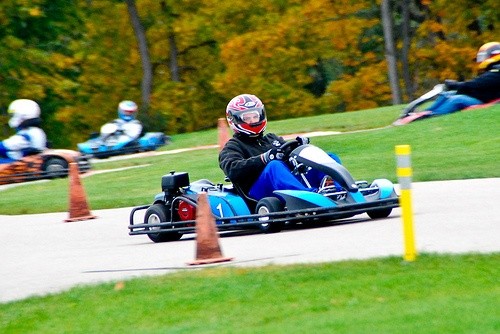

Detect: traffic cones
63;162;97;222
186;191;233;265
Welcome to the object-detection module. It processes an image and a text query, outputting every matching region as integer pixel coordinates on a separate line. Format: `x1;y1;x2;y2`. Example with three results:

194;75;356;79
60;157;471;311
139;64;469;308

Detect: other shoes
318;175;338;201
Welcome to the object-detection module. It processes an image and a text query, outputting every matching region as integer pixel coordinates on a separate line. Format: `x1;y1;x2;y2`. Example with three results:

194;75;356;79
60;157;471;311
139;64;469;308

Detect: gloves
445;79;457;89
296;136;310;145
263;147;285;163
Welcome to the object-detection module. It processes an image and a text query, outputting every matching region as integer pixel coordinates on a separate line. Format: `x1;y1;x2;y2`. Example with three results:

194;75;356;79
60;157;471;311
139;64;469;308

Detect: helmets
477;41;500;75
225;94;267;135
7;98;41;128
118;100;139;121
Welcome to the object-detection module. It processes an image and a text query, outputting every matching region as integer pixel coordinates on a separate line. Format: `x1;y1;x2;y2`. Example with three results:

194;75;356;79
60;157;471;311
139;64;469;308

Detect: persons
98;100;141;142
426;42;500;117
0;99;46;164
218;94;367;213
